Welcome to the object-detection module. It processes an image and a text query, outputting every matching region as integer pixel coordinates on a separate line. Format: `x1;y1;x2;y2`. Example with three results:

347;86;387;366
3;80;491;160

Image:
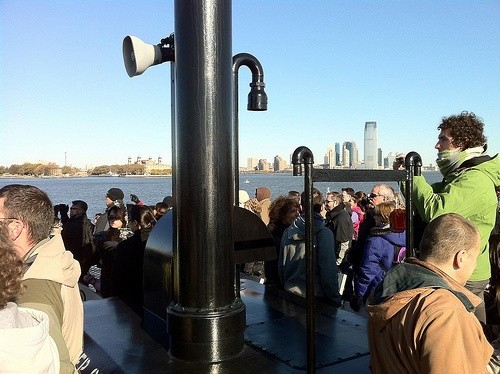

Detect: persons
393;110;500;326
365;213;495;374
0;220;61;374
52;182;410;310
0;184;84;374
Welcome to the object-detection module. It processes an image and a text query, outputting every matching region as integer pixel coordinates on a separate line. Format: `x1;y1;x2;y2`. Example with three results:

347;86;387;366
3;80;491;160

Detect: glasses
373;213;380;216
105;196;108;197
370;193;383;198
326;200;332;203
71;207;76;210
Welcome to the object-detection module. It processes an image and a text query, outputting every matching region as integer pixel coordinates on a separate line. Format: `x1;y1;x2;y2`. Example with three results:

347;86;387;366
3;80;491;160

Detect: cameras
396;153;406;170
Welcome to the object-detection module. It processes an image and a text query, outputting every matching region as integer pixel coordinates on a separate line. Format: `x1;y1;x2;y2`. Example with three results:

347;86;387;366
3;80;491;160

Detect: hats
239;190;249;203
164;196;172;206
107;188;124;201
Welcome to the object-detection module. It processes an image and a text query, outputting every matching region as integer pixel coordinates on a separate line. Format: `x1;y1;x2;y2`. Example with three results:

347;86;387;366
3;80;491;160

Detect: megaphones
122;35;174;77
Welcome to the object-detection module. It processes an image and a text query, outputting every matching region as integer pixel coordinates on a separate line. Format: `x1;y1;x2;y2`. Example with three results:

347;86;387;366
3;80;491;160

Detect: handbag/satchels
83;265;101;292
